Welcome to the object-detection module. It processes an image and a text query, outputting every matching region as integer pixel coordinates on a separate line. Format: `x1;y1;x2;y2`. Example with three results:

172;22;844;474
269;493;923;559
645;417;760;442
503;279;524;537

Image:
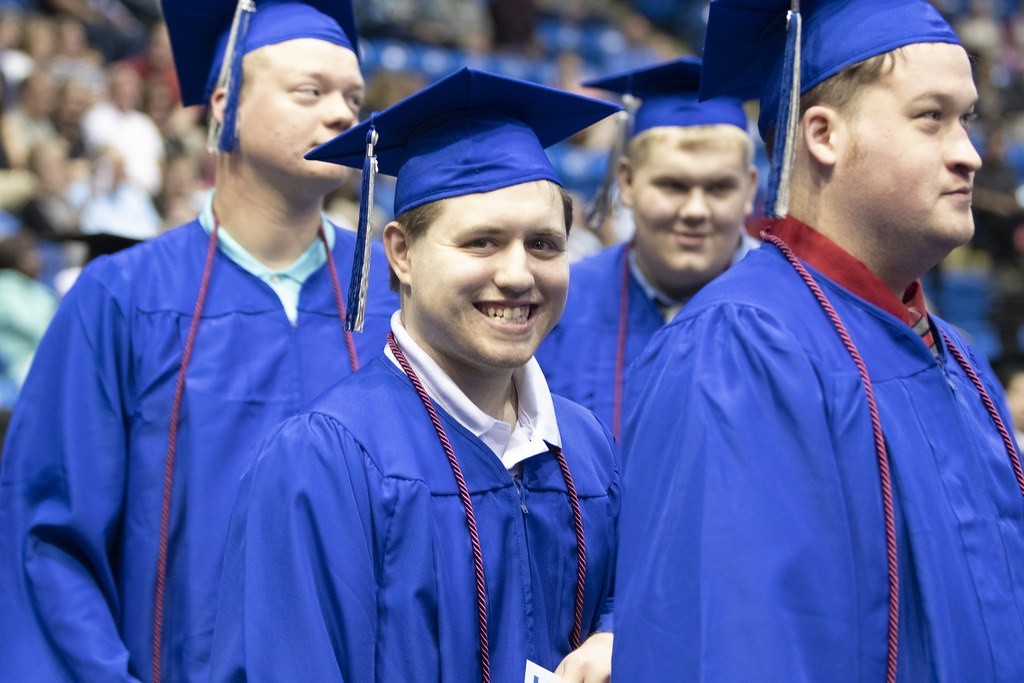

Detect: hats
695;0;969;139
303;69;627;219
157;2;359;106
583;57;757;133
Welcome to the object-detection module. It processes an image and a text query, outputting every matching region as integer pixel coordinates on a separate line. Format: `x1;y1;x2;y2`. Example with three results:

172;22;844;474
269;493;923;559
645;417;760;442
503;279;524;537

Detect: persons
203;69;625;683
0;2;1024;436
613;0;1024;683
2;0;405;682
537;59;774;442
